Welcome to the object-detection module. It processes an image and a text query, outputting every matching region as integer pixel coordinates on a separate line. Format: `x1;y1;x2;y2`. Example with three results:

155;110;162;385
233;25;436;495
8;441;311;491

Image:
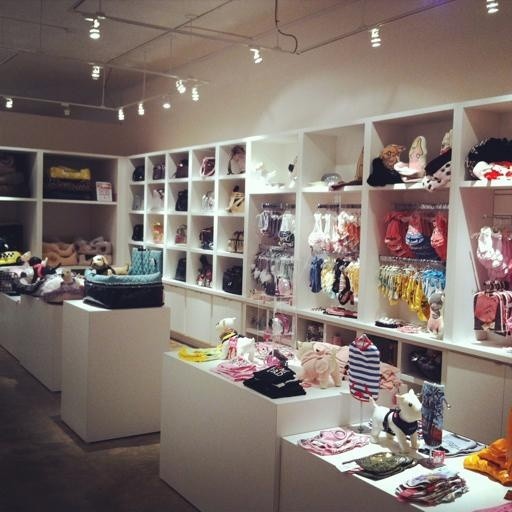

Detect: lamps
1;14;212;121
71;0;512;63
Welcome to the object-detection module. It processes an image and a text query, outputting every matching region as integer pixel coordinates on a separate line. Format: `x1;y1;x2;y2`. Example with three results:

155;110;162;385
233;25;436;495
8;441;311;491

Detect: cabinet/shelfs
0;260;62;394
281;416;512;512
247;96;512;443
59;299;171;443
157;349;408;512
125;139;247;350
0;147;125;271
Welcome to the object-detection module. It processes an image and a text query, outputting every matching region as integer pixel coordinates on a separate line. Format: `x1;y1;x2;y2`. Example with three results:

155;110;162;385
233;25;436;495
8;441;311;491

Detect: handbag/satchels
132;152;245;295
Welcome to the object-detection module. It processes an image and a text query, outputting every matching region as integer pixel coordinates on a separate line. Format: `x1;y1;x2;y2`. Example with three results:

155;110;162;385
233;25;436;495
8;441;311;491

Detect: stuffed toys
292;338;344;390
365;388;425;454
59;268;81;289
27;254;61;285
215;316;258;364
88;252;117;276
